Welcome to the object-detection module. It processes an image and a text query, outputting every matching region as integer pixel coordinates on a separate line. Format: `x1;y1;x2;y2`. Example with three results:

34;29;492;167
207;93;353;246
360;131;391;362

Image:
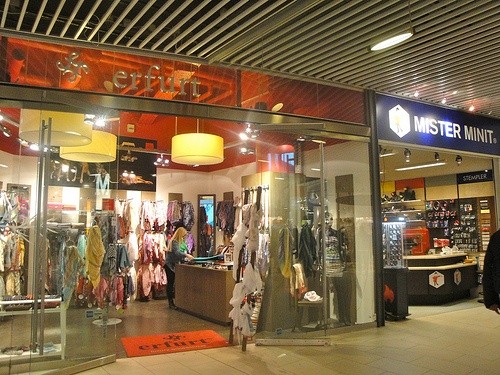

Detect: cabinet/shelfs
0;298;66;360
381;199;425;223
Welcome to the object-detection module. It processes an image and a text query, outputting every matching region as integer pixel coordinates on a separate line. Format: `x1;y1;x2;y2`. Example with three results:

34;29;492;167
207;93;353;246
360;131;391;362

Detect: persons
163;227;194;309
482;229;500;314
315;213;352;328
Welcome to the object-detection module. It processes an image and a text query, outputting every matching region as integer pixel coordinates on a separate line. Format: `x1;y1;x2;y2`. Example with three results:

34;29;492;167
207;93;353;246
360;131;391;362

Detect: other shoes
169;300;176;309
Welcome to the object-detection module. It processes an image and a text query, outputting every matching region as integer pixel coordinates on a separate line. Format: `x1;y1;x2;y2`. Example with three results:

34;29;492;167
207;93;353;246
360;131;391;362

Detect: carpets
120;329;230;357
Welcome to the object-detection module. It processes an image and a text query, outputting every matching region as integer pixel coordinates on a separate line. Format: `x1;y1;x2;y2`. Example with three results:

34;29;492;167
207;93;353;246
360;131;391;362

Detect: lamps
171;119;224;166
434;152;440;161
19;108;93;147
456;155;462;166
59;130;117;163
403;147;411;162
